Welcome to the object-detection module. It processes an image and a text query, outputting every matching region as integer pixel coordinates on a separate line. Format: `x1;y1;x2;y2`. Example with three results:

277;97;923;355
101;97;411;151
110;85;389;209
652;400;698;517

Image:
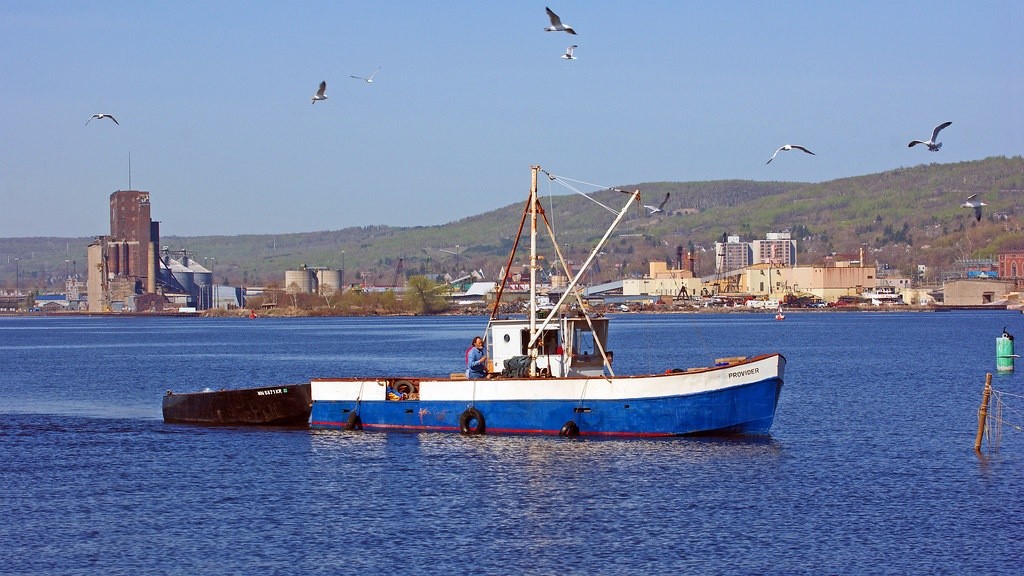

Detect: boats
161;163;787;440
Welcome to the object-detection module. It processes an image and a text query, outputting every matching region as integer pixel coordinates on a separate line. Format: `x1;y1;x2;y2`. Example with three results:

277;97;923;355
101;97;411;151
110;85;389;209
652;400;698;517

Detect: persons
466;336;488;378
549;336;558;354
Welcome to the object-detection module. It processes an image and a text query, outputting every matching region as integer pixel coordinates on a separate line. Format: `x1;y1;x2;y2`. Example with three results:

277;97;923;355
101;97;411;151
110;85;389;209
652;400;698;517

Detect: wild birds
543;6;578;35
560;44;578;60
84;114;119;127
908;122;952;152
311;80;327;104
766;144;815;164
960;194;987;222
643;192;670;215
350;73;376;83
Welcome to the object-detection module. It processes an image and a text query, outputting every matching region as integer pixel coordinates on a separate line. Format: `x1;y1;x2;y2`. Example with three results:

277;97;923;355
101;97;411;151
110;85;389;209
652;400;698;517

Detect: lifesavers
394;380;414;395
460;409;484;434
560;421;573;436
